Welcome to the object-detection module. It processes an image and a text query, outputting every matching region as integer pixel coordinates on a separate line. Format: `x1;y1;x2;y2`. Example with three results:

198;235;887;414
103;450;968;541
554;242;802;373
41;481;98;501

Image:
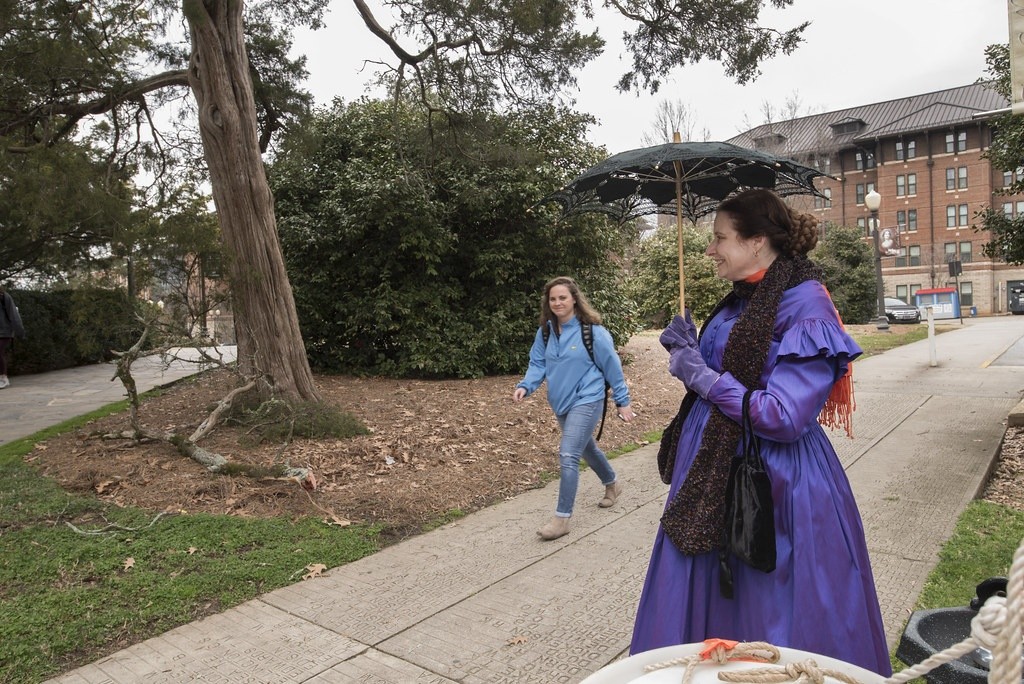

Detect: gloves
667;330;721;401
659;309;698;353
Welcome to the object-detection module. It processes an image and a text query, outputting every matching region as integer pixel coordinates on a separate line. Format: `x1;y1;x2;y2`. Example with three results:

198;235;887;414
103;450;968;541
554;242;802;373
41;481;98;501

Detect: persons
628;189;893;679
511;275;634;539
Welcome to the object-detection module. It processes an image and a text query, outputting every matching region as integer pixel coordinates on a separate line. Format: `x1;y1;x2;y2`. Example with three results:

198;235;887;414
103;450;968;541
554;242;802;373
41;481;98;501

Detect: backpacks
542;322;611;390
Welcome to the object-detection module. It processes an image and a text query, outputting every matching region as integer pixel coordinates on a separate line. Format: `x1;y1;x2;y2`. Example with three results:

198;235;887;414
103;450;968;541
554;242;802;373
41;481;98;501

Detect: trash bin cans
970;306;978;317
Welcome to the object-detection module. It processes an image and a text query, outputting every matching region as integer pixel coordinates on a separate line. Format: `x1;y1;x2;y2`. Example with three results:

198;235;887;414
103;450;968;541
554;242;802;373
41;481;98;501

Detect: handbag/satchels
723;389;777;574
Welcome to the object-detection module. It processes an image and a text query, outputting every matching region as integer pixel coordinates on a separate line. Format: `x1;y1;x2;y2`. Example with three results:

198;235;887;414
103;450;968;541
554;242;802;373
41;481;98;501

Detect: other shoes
599;481;622;507
536;515;571;539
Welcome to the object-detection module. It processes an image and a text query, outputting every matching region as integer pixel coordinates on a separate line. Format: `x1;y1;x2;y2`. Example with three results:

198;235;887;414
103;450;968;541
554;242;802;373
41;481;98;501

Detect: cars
876;298;921;324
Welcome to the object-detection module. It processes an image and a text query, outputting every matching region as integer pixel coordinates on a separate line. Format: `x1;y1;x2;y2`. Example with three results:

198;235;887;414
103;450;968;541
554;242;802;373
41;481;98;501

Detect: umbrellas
526;130;843;327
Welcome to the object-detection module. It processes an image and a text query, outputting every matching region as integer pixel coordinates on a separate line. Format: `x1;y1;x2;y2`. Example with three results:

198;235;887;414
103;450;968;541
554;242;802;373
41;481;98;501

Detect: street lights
865;189;889;330
